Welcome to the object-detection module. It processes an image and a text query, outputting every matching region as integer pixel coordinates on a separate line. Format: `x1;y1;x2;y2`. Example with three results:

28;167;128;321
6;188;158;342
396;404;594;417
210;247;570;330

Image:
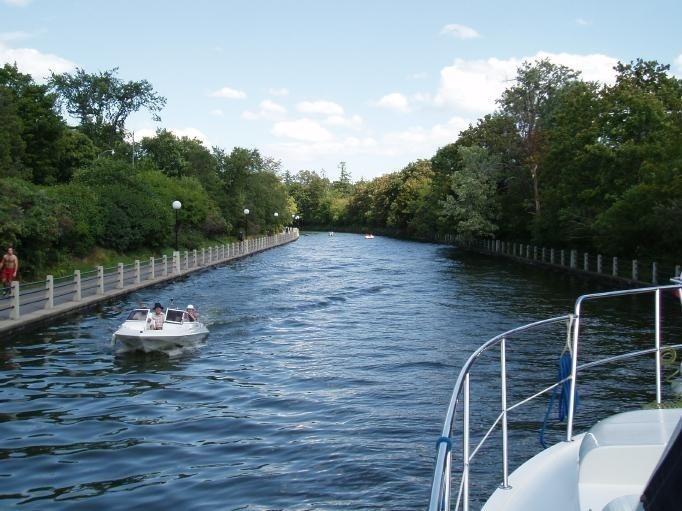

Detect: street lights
290;215;300;232
172;201;181;250
243;208;250;240
273;213;279;235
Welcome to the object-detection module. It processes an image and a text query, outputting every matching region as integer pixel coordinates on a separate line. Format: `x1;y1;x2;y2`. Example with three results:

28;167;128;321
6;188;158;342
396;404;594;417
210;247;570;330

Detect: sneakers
2;291;7;296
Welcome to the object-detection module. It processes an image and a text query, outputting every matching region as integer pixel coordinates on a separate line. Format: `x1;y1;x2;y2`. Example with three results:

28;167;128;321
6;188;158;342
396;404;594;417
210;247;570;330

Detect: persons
0;246;19;299
135;304;156;331
184;304;198;322
151;303;166;331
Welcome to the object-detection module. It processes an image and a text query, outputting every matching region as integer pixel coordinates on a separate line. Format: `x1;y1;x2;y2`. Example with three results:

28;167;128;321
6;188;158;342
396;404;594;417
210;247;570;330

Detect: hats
152;303;164;310
185;304;195;310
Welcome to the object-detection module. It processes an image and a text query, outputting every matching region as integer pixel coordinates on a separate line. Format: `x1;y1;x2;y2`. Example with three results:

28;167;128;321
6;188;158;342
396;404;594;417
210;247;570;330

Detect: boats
427;276;682;510
364;234;374;240
112;299;210;355
328;232;336;236
